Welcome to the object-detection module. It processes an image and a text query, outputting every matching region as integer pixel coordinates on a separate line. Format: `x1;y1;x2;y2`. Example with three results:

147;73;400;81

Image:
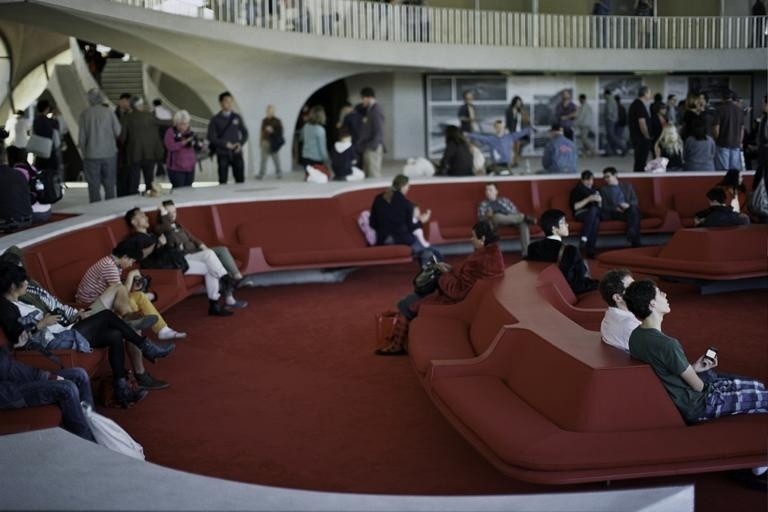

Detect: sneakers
224;300;248;310
158;330;186;340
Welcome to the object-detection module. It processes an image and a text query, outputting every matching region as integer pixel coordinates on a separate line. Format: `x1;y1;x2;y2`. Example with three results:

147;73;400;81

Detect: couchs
0;171;768;436
594;223;766;299
403;259;766;485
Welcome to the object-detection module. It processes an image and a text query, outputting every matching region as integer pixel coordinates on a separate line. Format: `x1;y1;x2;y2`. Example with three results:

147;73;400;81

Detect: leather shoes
376;347;408;355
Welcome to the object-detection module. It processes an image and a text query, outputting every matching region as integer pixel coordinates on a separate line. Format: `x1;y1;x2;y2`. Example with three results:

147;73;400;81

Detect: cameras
701;347;717;366
51;307;70;327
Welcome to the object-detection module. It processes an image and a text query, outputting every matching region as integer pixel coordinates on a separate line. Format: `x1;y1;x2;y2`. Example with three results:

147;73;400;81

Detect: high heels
135;335;176;364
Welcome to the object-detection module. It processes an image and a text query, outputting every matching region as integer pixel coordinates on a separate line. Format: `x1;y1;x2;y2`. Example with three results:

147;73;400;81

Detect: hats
2;243;26;269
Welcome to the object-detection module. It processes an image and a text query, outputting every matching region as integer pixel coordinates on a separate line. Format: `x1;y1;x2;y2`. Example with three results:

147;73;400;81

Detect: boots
219;274;250;296
132;369;170;390
108;376;149;408
207;299;234;317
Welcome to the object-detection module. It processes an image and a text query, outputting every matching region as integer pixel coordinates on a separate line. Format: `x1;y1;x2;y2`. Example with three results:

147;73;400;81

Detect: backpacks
748;162;767;217
25;134;53;159
466;142;485;174
32;167;63;204
207;141;217;161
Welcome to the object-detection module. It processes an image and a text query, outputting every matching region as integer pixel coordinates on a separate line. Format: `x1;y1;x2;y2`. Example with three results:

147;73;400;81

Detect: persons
569;170;603;260
711;89;746;171
622;278;768;475
646;93;663;116
0;344;95;444
597;268;644;356
163;110;196;188
677;100;685;120
575;93;599;159
535;123;577;174
612;94;629;157
713;168;750;226
0;145;34;233
207;91;248;185
0;265;174;408
354;102;368;123
116;206;250;316
529;208;599;293
733;96;746;170
694;188;749;227
654;124;685;173
433;125;473;176
358;86;389;179
150;201;251;310
476;182;537;260
698;92;716;121
4;144;65;226
121;94;172;194
370;175;432;249
599;166;642;249
300;105;331;181
550;89;579;141
1;244;171;390
336;101;361;177
750;153;767;224
372;220;506;357
504;95;539;169
666;93;679;125
149;98;169;177
681;90;708;140
649;102;669;143
462;120;538;176
27;96;61;176
10;109;30;151
113;92;130;197
457;90;483;132
78;87;122;205
683;118;717;173
627;87;655;172
254;104;283;181
755;93;767;161
600;88;629;158
74;239;187;341
